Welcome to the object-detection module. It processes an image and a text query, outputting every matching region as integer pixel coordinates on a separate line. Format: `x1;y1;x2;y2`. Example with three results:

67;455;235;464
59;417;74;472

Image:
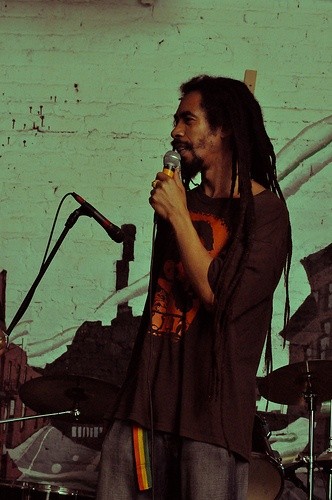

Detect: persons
94;68;292;500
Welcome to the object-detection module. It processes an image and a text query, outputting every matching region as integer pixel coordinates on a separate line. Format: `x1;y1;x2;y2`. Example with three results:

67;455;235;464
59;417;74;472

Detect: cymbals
260;359;332;405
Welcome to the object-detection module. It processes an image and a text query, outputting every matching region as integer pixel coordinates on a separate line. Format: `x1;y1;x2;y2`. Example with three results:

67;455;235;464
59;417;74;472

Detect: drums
0;481;96;500
245;452;285;500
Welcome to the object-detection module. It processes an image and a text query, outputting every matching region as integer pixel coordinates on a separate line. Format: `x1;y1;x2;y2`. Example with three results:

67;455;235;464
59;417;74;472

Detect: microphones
71;192;124;243
154;151;181;223
